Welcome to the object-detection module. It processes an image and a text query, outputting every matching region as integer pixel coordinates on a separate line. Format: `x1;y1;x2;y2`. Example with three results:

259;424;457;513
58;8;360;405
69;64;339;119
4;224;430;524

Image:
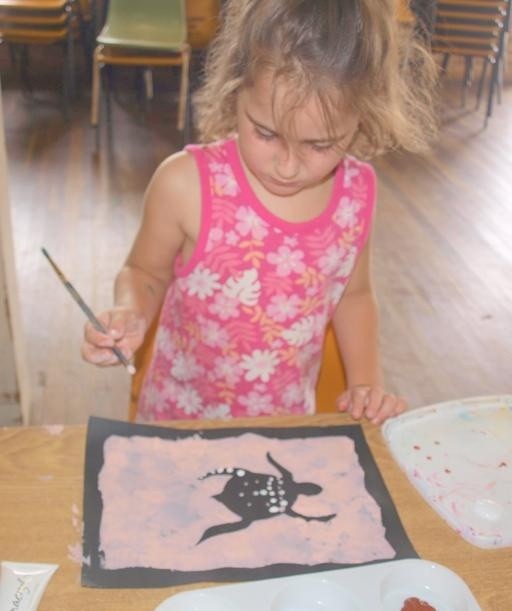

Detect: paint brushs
42;248;136;376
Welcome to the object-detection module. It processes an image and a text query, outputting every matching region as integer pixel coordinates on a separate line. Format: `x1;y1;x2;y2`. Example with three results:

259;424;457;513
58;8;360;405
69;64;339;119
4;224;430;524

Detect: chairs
87;2;194;130
186;1;218;54
421;2;511;129
385;4;414;60
1;1;90;122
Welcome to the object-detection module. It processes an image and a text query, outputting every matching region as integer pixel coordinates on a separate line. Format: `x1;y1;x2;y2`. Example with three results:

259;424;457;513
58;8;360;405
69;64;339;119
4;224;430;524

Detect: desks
0;408;511;611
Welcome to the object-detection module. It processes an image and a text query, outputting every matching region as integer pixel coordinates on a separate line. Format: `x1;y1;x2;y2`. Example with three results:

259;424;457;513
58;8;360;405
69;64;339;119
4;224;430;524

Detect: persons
78;2;432;425
